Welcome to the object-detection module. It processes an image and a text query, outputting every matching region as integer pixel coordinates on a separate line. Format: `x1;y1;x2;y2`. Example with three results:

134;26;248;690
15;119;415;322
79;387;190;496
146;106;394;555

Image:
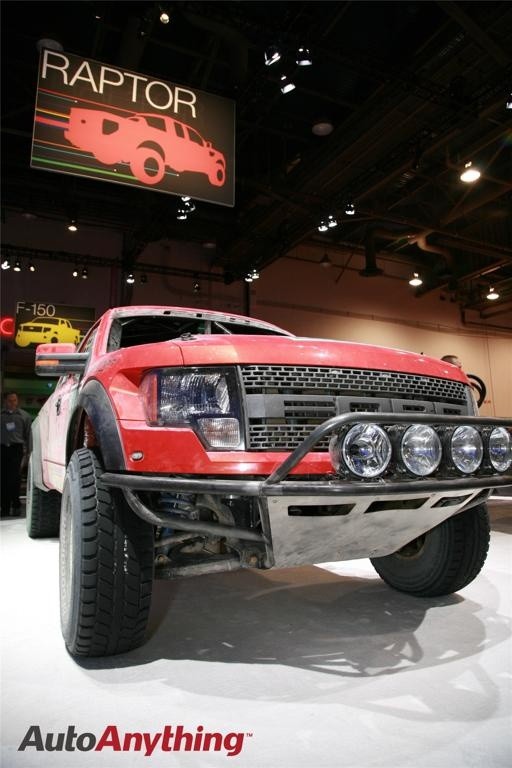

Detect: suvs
26;305;512;658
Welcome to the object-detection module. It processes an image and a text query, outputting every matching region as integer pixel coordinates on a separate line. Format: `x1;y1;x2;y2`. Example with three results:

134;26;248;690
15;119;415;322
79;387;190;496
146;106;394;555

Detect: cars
14;317;81;347
64;106;227;188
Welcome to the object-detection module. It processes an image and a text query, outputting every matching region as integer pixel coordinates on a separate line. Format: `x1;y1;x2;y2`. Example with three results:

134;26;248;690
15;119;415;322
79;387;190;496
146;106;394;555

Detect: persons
440;355;462;369
0;390;33;516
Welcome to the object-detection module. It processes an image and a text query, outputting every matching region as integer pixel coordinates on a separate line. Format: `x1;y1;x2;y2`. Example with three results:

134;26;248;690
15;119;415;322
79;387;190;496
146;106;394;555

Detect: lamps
0;195;227;292
